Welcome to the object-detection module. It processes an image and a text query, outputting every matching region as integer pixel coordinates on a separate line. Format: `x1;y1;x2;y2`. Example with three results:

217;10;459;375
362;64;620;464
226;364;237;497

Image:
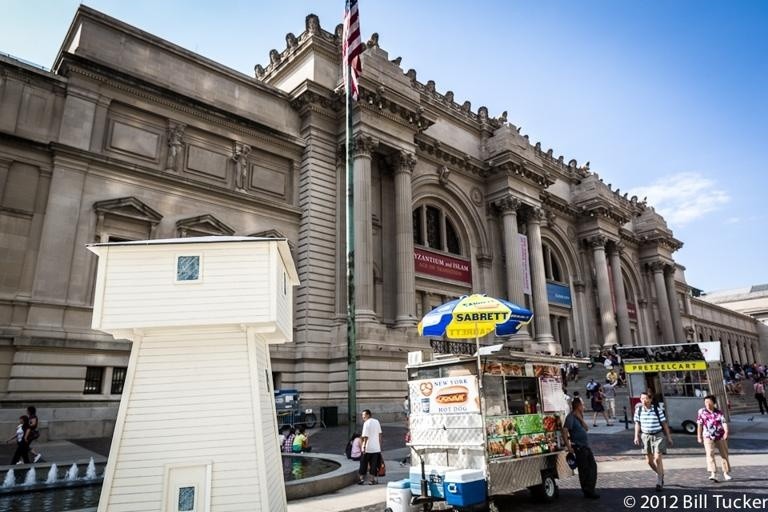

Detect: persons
537;344;768;428
357;409;386;484
403;395;410;423
345;433;357;459
231;144;253;194
12;405;42;465
632;392;673;492
292;426;312;453
281;427;295;453
351;432;364;460
279;427;287;453
6;415;41;465
563;397;600;500
166;124;186;176
696;395;733;482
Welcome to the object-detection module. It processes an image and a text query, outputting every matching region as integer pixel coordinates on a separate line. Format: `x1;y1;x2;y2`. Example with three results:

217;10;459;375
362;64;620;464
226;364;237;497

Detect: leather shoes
656;485;661;491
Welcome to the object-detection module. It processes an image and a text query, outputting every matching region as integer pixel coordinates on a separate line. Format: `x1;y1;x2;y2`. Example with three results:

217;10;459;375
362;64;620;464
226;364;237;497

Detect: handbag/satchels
27;431;39;439
375;464;376;472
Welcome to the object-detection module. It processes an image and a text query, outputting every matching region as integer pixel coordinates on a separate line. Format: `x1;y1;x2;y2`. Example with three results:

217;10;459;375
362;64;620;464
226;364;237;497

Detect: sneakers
582;490;600;498
16;453;41;465
725;474;734;480
709;473;718;480
593;424;613;427
358;479;377;484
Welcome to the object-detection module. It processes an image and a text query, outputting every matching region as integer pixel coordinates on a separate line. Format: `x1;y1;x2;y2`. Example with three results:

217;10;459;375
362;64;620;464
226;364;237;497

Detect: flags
342;1;365;102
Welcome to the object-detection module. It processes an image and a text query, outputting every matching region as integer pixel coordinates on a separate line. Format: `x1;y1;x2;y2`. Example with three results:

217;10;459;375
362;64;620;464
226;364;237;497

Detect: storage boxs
443;469;486;507
409;465;454;499
386;479;425;512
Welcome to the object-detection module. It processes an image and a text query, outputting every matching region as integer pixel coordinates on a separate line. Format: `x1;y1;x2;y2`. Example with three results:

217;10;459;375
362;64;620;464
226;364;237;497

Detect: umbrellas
416;294;532;386
